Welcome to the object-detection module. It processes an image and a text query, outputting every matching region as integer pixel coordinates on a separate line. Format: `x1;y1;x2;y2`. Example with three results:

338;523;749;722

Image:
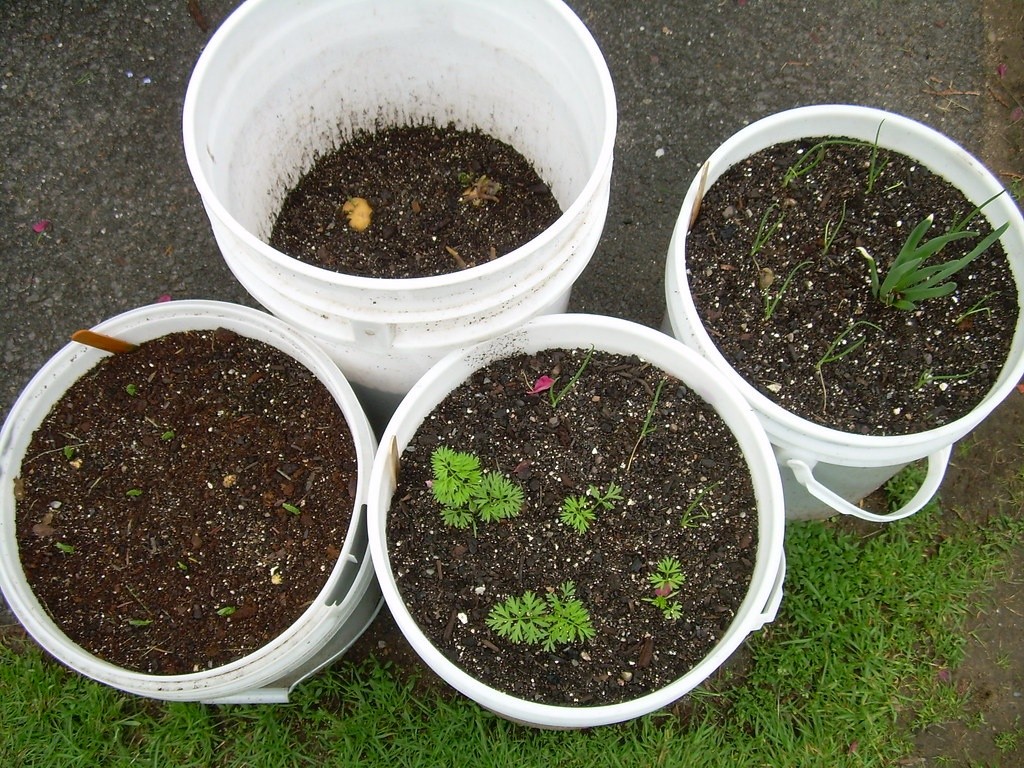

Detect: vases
0;297;380;705
182;2;620;410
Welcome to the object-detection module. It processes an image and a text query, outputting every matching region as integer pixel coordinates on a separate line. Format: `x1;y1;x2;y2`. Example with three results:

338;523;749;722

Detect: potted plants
659;104;1023;520
371;314;786;732
